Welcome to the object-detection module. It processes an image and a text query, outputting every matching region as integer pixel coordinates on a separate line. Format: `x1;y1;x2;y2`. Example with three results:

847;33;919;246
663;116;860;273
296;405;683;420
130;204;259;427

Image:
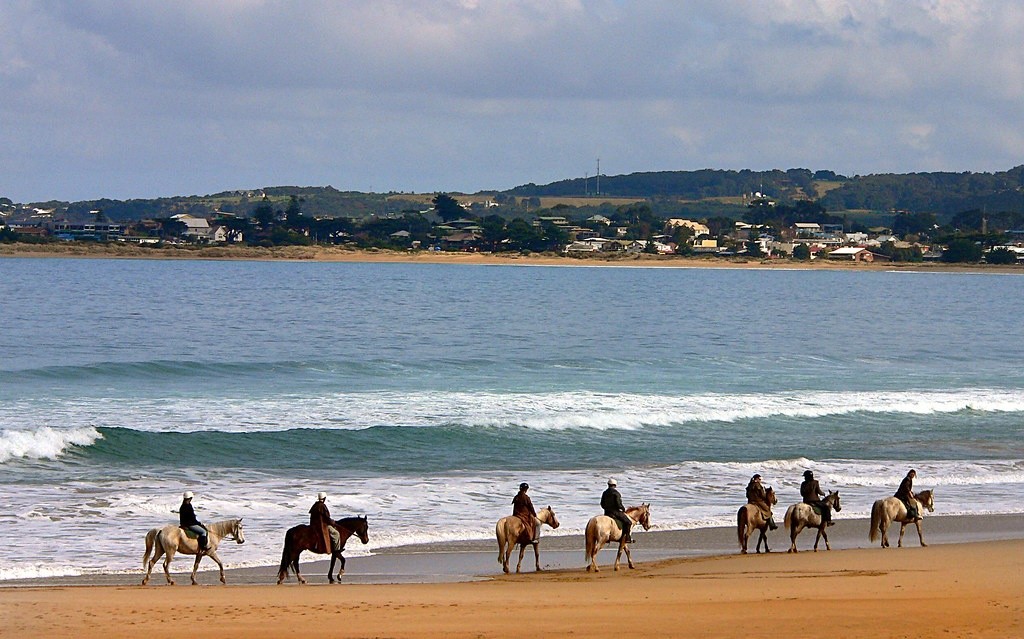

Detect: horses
736;485;777;554
495;506;560;575
277;514;369;585
142;517;246;586
584;502;652;573
867;488;936;548
784;489;842;554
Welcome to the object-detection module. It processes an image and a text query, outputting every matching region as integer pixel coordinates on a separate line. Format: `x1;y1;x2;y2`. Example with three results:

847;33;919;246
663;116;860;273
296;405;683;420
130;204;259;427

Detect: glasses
759;479;761;481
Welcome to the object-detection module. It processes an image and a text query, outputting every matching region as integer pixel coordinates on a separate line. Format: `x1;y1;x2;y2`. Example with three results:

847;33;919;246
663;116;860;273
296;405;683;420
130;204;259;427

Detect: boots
768;517;778;530
200;536;211;551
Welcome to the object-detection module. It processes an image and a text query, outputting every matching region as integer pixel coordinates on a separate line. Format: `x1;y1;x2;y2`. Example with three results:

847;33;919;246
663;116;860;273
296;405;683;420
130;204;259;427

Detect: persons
745;474;778;530
179;490;211;551
511;483;539;544
601;478;636;543
309;492;345;557
894;469;924;521
801;470;835;527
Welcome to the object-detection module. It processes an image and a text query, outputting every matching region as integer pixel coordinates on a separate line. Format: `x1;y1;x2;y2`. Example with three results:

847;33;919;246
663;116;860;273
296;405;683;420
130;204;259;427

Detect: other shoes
828;522;835;527
533;539;539;543
625;535;635;543
334;548;345;555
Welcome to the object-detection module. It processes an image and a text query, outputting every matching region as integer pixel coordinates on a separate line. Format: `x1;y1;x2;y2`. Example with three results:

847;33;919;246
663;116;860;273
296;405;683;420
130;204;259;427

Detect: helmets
608;478;617;486
520;483;529;489
184;491;194;498
318;492;326;499
802;471;813;477
